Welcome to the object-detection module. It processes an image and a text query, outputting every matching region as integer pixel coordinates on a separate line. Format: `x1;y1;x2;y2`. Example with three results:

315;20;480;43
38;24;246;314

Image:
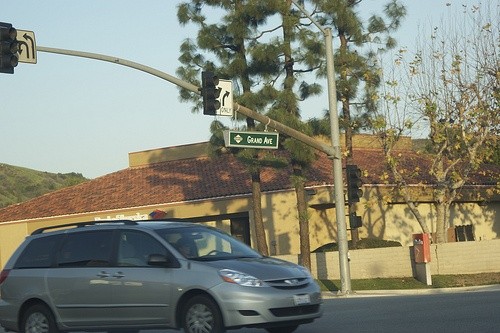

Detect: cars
0;218;324;333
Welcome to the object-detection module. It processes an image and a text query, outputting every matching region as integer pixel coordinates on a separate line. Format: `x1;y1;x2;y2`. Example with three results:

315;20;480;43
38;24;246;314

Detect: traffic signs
229;131;279;150
214;79;234;116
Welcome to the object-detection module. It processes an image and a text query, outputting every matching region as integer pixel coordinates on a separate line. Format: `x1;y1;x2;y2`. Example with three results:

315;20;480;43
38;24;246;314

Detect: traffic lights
201;71;221;115
0;22;19;74
349;214;362;228
346;165;363;202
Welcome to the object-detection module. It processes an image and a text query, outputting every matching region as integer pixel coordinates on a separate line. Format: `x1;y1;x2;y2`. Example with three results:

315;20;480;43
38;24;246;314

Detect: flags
149;207;167;219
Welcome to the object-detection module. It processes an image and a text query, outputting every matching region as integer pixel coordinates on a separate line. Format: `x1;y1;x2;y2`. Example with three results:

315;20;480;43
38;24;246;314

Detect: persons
167;236;199;266
